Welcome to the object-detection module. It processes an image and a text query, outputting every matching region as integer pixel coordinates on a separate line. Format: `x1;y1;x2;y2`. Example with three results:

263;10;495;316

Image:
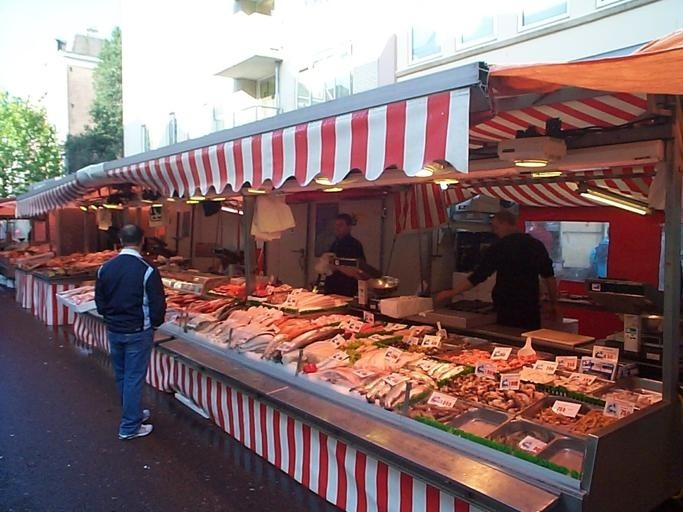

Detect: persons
94;224;167;441
323;213;366;298
435;210;559;330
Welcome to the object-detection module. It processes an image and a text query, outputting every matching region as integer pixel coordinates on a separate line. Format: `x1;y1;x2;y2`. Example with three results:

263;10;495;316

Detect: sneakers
142;410;150;422
118;423;153;440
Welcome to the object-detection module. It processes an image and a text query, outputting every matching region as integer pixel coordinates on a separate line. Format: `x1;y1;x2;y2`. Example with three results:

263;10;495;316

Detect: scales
211;249;243;279
334;257;403;315
604;315;682;370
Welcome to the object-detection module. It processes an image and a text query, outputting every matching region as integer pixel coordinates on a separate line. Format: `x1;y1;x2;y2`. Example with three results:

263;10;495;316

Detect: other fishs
164;284;466;423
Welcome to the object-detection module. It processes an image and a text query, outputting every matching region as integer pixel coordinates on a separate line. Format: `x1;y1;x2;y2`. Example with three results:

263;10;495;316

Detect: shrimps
441;349;545;372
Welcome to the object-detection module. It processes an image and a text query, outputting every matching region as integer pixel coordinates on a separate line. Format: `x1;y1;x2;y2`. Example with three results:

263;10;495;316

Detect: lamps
497;135;567;168
530;171;562;179
102;183;136;210
416;161;443;177
90;202;99;210
313;174;337;186
574;180;652;216
141;189;161;203
80;205;89;212
433;178;459;190
